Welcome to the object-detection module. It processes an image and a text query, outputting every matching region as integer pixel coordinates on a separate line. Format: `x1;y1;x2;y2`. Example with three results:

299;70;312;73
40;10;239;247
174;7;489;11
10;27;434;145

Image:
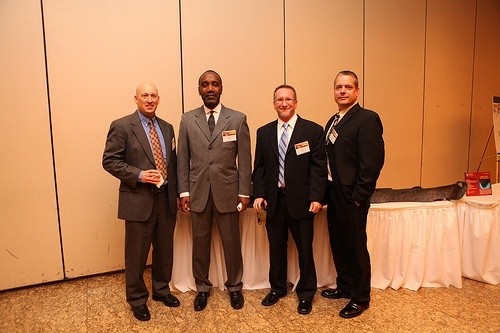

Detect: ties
147;118;168;181
277;123;290;187
208;111;215;137
328;113;340;141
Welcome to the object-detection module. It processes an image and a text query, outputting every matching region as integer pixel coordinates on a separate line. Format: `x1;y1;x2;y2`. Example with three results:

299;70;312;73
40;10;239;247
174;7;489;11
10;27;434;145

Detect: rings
316;210;319;212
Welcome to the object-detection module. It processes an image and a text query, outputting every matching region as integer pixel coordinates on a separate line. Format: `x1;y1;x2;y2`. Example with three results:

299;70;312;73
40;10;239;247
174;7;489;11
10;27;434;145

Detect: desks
457;183;500;284
169;199;463;293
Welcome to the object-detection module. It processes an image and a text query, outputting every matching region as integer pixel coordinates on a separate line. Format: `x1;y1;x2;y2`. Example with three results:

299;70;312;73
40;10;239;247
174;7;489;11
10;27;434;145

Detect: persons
251;85;328;314
177;70;250;310
102;83;181;321
320;71;385;318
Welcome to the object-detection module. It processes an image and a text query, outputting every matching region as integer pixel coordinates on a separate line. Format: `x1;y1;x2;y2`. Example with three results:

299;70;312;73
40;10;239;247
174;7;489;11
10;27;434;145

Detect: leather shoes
130;302;151;321
230;290;244;309
151;292;180;307
297;299;312;314
320;288;352;299
339;299;370;318
261;290;288;306
194;291;211;311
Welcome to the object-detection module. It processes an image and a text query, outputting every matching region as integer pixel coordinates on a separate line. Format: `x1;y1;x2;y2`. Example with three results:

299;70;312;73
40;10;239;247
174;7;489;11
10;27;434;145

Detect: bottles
257;202;266;225
